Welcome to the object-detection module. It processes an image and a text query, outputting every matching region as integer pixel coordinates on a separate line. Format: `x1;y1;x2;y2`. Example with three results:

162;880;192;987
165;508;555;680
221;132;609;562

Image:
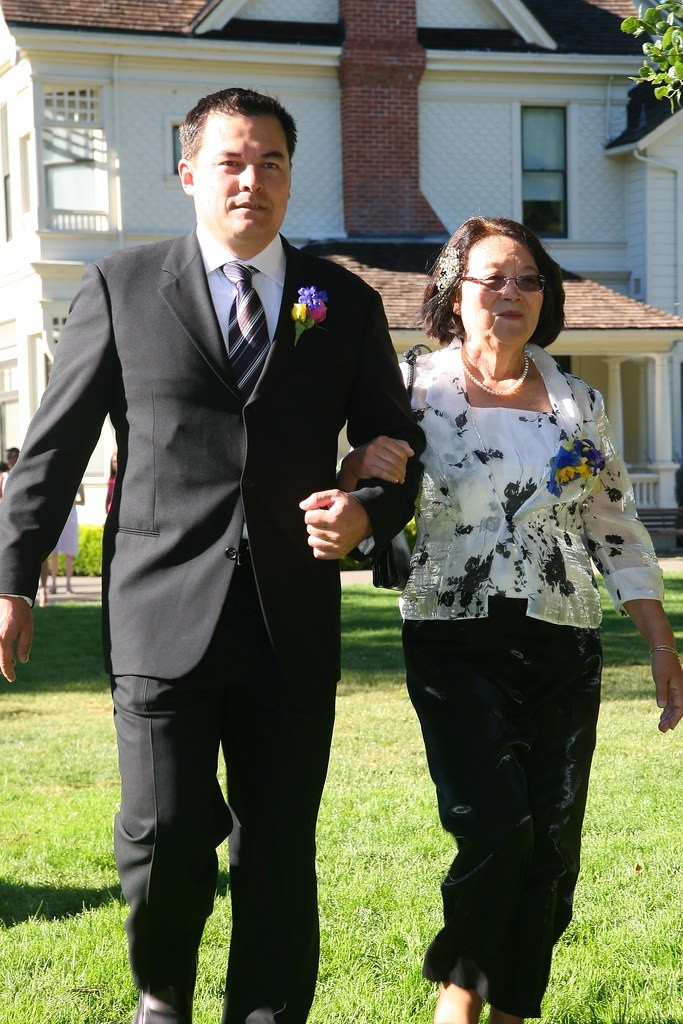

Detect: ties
220;262;271;402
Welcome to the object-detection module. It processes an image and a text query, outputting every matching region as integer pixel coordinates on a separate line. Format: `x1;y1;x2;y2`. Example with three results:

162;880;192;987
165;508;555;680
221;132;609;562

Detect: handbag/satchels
372;343;433;591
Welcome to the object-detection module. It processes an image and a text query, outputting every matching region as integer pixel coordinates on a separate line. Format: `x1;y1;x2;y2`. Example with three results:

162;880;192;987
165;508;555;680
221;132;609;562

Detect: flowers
291;284;329;345
547;437;607;499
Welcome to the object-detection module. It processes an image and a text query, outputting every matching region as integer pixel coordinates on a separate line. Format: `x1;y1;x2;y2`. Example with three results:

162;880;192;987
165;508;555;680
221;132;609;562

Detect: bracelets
647;645;681;657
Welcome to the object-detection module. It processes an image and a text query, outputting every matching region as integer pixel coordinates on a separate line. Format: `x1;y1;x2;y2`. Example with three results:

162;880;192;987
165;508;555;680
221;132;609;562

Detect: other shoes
49;586;57;594
132;990;185;1024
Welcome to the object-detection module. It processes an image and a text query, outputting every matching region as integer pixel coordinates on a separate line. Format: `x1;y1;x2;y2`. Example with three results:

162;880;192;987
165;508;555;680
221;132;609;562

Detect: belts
237;541;250;571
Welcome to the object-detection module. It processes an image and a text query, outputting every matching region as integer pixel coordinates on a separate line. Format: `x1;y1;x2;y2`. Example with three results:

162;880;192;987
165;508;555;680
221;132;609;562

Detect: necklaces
452;348;540;398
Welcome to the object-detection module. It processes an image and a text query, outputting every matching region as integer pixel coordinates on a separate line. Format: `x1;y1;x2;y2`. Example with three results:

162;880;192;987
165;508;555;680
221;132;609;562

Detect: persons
337;215;683;1023
4;87;427;1023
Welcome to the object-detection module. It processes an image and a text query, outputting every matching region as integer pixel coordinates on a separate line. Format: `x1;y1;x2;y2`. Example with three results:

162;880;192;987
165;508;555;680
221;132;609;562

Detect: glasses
460;275;545;292
